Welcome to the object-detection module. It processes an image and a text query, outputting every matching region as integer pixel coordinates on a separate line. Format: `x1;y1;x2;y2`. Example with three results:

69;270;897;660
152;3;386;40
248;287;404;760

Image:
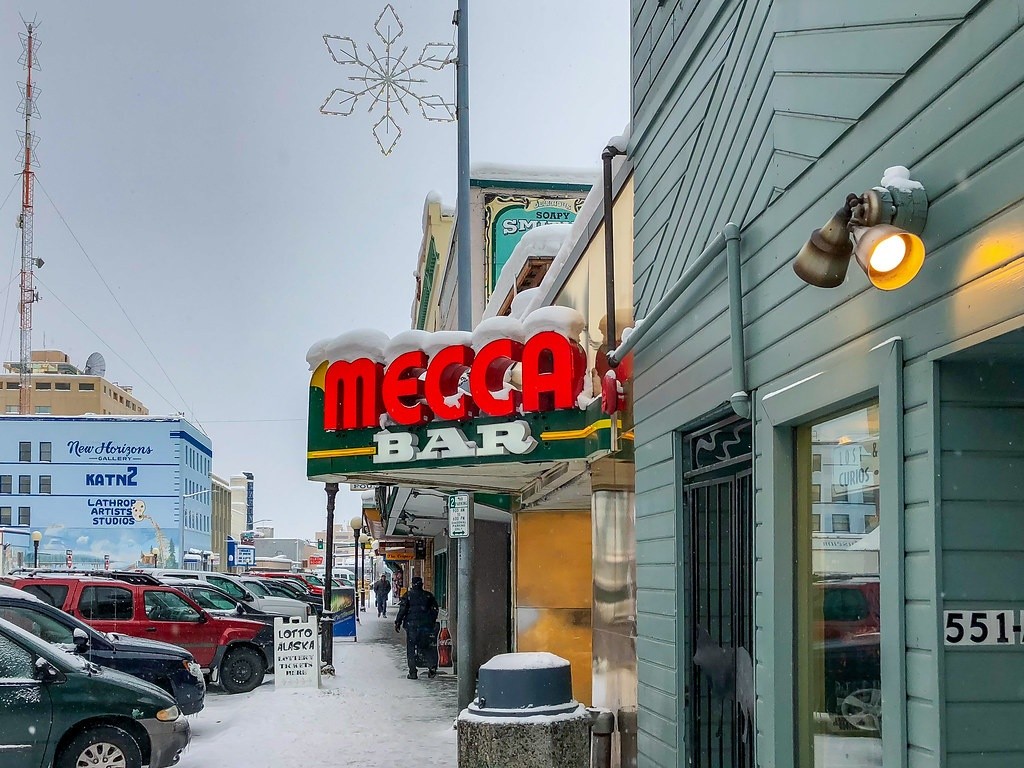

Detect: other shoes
378;612;380;617
407;673;417;679
428;664;436;678
383;615;386;618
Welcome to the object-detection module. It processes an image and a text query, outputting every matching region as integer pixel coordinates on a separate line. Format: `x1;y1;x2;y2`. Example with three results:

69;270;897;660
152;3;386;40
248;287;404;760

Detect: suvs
0;567;272;694
811;571;882;738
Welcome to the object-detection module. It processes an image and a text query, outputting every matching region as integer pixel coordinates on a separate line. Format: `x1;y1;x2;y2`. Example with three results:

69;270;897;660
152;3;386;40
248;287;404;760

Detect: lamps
793;182;927;292
400;511;416;522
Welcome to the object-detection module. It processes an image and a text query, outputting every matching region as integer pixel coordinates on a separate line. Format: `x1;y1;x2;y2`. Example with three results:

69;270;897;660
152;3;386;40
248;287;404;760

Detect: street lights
350;515;363;621
359;533;368;611
151;547;159;567
180;489;221;570
30;530;42;567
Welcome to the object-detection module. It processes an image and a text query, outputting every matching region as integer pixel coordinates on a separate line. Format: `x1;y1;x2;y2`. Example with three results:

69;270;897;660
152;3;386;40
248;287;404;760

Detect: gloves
395;620;401;633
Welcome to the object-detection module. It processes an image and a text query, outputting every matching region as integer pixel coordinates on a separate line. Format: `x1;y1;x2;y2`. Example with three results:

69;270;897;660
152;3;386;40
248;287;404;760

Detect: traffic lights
317;539;323;549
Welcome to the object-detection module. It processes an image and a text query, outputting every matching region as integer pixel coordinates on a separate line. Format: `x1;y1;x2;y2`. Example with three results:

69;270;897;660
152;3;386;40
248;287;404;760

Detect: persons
373;575;391;617
395;577;439;679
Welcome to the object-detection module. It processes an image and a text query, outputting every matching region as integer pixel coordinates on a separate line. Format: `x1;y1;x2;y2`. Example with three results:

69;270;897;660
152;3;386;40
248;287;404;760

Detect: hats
411;577;422;584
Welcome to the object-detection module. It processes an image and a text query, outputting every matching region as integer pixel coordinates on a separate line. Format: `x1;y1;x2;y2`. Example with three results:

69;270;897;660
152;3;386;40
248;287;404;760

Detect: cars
0;585;206;717
53;567;362;673
0;617;192;768
